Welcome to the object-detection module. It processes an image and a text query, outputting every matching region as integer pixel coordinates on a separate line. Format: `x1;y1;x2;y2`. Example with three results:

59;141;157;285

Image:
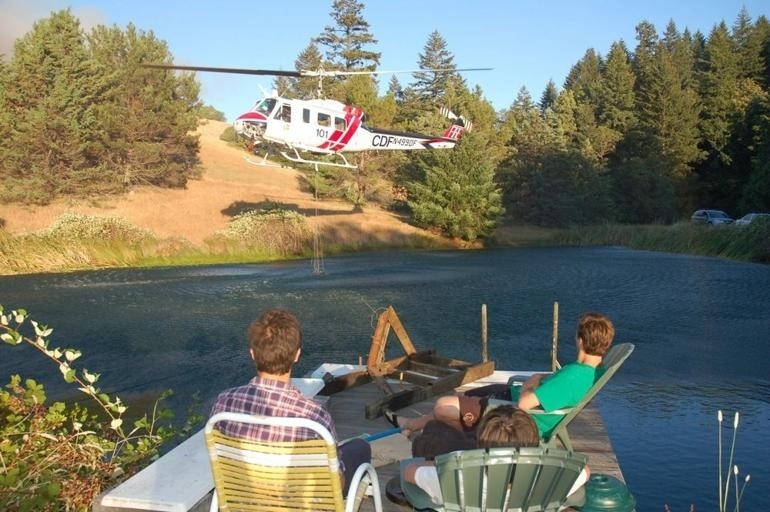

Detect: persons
403;404;590;507
207;308;371;512
382;312;615;440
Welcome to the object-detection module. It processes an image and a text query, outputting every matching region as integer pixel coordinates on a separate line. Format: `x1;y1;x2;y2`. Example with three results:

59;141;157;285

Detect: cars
735;213;769;225
691;209;736;227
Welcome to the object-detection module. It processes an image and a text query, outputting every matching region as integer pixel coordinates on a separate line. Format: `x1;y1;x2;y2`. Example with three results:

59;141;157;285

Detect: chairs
399;447;589;512
204;412;383;512
482;342;635;452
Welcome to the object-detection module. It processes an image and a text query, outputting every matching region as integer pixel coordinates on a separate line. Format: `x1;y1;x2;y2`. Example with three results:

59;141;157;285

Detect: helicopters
137;65;495;170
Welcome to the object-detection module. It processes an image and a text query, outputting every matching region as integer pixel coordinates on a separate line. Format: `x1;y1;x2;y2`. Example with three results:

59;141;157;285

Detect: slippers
382;408;400;428
